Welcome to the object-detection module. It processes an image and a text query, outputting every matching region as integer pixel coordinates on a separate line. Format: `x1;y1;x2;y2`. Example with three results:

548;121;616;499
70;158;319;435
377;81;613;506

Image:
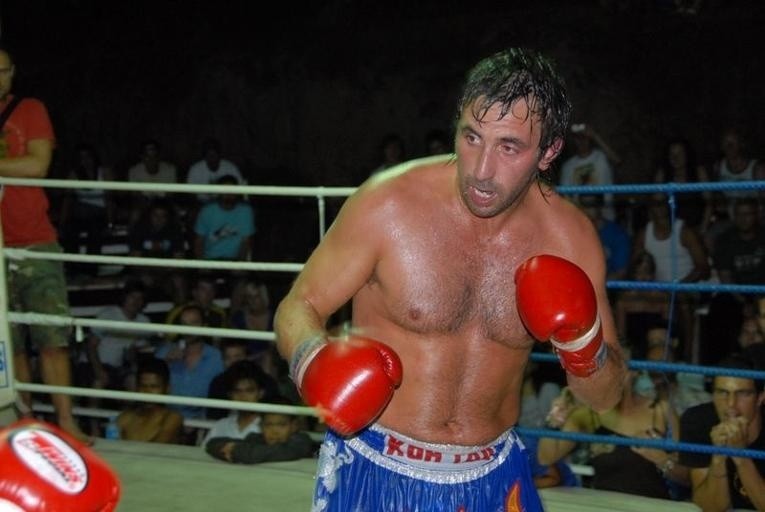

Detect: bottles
105;415;122;440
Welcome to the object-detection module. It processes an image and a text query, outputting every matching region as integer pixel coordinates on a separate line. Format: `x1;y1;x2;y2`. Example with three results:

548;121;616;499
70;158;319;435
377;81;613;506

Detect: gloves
0;415;122;512
512;253;608;380
281;331;406;443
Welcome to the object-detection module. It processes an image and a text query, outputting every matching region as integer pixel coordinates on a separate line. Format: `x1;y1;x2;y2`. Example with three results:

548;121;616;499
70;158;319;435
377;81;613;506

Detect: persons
0;46;104;447
520;122;765;511
273;46;630;512
69;134;450;465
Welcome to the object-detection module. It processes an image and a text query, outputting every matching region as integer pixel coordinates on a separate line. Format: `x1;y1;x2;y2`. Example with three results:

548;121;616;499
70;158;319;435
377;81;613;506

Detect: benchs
27;223;232;366
